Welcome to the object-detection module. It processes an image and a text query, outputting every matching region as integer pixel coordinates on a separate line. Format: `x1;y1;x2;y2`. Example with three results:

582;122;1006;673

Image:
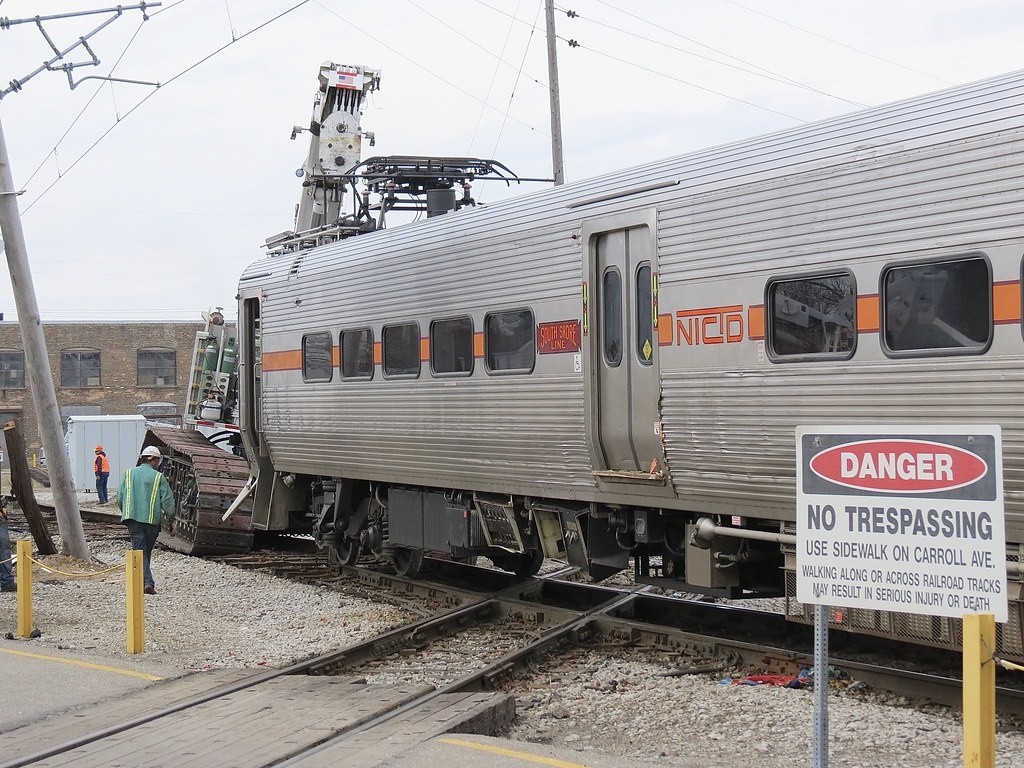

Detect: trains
220;59;1024;681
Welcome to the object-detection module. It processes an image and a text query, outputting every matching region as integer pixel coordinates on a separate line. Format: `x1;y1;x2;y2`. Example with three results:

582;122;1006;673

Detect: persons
94;445;110;504
115;446;175;594
94;445;111;504
0;503;17;592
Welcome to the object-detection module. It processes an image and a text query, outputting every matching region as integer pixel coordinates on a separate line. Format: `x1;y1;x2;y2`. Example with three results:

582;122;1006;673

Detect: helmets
94;446;103;452
139;446;162;460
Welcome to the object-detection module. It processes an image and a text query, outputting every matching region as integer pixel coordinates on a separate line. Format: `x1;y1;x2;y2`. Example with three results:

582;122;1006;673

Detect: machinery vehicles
133;303;263;558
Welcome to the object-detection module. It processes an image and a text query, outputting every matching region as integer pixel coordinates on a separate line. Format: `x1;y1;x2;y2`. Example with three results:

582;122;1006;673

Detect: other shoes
144;584;156;594
96;500;108;504
